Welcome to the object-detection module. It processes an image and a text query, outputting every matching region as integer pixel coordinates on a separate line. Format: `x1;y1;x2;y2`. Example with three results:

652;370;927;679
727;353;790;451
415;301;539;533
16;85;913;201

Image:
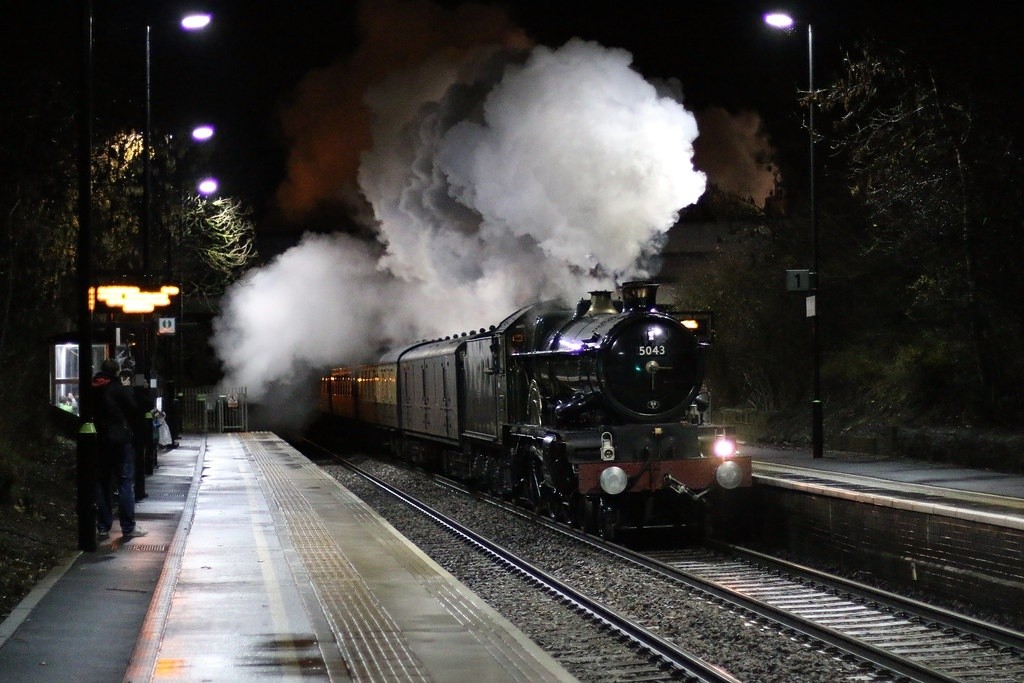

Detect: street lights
144;10;213;294
173;123;218;441
762;7;825;459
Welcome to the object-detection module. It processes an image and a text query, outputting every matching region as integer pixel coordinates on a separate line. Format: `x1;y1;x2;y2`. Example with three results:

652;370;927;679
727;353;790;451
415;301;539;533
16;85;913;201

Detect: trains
311;279;753;549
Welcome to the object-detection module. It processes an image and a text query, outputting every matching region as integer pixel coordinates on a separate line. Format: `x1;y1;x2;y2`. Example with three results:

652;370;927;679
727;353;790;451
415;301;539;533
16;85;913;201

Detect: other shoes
99;529;109;535
123;527;149;537
136;494;149;503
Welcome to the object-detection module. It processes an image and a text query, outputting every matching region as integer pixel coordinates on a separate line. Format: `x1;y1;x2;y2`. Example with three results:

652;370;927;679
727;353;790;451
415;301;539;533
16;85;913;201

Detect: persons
90;356;155;536
